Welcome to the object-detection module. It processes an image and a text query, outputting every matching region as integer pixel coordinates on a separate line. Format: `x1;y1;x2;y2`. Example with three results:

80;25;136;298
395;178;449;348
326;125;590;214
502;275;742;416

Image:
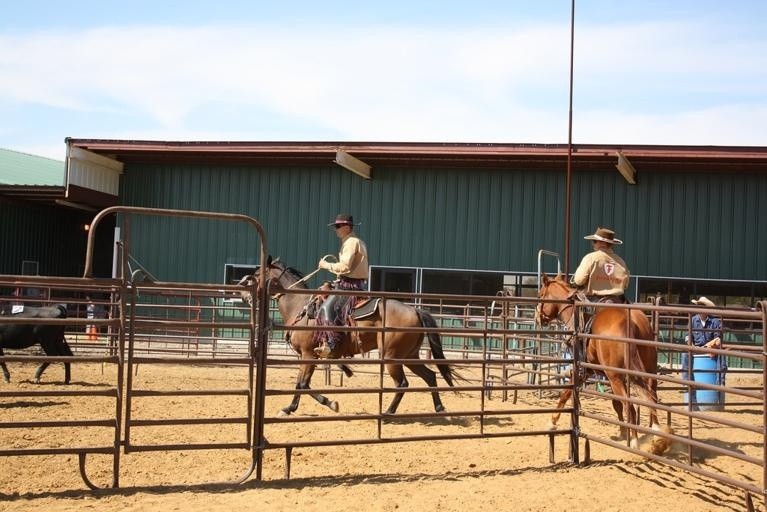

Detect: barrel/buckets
681;354;727;412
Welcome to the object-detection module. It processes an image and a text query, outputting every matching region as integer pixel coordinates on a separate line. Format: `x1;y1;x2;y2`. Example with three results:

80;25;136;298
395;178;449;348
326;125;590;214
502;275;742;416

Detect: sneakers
314;346;335;354
678;385;695;393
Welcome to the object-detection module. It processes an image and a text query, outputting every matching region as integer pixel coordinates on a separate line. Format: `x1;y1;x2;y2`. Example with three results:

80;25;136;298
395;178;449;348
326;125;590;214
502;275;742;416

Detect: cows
1;304;77;385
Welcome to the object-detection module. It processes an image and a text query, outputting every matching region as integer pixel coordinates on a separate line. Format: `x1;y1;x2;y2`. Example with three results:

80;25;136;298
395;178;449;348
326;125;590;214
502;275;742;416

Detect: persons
675;295;726;392
307;214;369;358
561;228;630;378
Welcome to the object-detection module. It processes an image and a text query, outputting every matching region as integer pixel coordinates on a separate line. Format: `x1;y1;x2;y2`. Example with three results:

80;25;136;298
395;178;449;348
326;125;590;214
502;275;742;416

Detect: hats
692;297;715;306
583;228;622;244
327;214;361;227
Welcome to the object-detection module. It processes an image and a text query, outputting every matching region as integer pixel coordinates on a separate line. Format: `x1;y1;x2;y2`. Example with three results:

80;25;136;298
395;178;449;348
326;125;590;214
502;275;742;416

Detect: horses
533;269;664;451
241;255;473;417
